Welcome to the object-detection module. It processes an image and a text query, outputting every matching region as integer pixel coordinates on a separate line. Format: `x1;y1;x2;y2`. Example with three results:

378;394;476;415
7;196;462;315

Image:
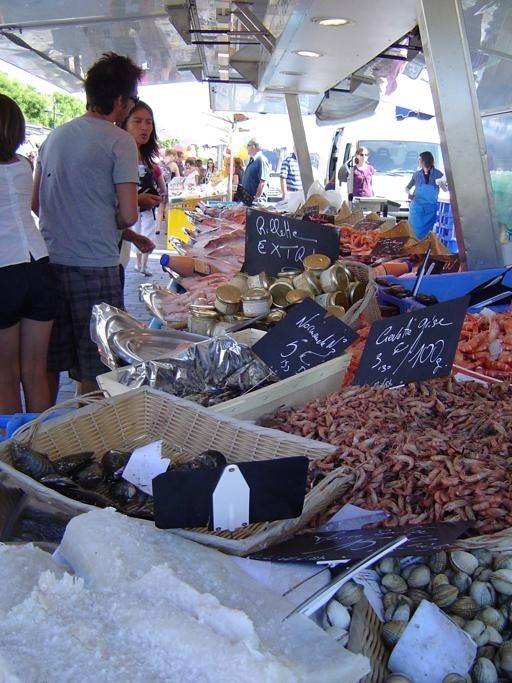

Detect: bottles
372;260;413;276
159;253;220;279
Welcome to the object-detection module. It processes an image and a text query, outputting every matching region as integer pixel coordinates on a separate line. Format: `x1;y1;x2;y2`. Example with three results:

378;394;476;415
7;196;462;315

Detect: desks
165;193;229;250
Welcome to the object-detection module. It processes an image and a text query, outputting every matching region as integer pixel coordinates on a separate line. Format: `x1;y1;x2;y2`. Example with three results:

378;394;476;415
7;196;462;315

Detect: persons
241;139;269;202
280;144;303;199
233;158;244;184
405;151;448;240
114;101;220;277
348;146;375;197
0;95;53;415
31;52;146;408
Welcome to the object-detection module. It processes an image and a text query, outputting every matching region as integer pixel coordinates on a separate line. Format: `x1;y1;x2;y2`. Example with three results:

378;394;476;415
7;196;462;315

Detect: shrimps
454;306;512;380
271;373;512;538
339;226;383;252
343;313;371;388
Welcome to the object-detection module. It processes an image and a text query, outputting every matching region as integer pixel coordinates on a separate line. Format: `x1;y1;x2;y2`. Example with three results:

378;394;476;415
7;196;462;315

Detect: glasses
360;154;368;156
129;95;139;106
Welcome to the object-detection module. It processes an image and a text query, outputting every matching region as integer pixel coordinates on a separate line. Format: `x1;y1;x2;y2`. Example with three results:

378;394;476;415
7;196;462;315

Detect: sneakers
135;264;152;276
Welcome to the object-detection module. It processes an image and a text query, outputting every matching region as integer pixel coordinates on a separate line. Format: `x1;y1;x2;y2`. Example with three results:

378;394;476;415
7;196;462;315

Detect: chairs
373;148;419;172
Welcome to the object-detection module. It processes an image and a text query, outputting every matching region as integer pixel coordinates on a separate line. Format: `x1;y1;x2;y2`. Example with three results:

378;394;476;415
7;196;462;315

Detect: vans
322;119;452;222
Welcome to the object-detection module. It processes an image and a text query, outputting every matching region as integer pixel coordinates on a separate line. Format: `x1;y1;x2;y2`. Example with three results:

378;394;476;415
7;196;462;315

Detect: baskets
335;208;363;224
345;529;512;682
0;385;355;557
